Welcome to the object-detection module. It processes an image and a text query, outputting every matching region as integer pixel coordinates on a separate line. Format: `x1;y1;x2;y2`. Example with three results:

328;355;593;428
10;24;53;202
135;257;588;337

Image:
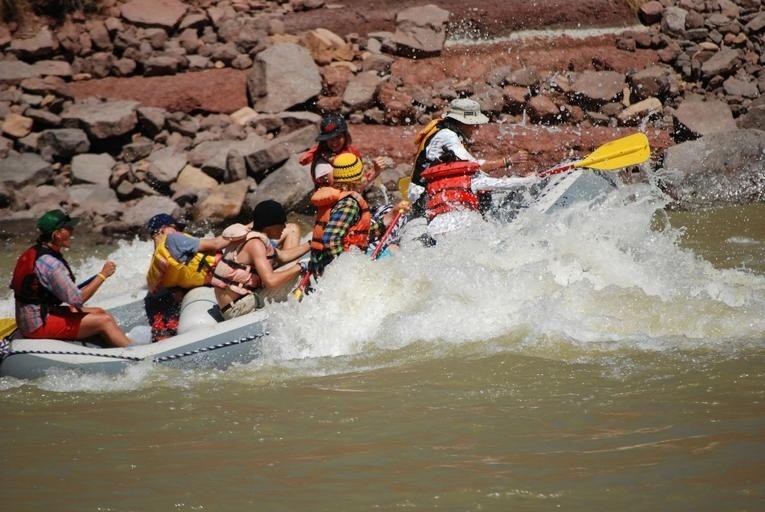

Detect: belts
221;294;246;314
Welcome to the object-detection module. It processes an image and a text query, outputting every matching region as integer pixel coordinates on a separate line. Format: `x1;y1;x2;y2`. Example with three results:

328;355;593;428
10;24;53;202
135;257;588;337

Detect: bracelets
97;272;105;280
503;156;512;169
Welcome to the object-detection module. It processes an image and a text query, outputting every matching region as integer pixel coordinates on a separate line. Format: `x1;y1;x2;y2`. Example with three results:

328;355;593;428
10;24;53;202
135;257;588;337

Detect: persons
302;153;410;270
407;98;529;199
12;210;134;355
211;201;316;322
145;211;302;295
310;113;391;191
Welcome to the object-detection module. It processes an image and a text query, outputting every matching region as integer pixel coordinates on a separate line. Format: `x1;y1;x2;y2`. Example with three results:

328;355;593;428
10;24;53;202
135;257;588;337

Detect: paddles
538;133;652;177
0;273;105;339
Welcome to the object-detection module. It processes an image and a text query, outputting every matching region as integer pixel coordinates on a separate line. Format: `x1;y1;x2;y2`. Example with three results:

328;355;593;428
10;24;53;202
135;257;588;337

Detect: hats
148;214;187;238
441;98;490;125
252;200;286;228
333;152;364;183
315;112;347;141
37;209;81;234
